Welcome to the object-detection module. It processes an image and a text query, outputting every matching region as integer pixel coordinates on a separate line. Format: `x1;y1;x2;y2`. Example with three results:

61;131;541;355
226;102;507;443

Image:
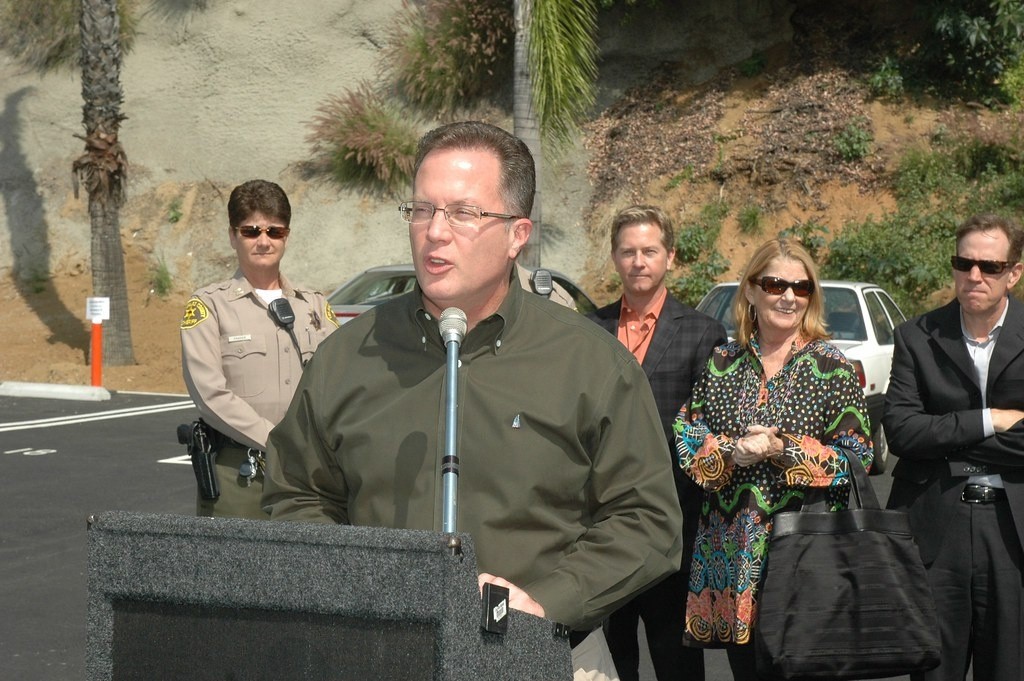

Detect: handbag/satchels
755;449;941;681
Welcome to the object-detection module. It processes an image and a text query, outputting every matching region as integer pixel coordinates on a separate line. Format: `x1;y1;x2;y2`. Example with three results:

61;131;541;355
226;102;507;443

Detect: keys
239;455;265;488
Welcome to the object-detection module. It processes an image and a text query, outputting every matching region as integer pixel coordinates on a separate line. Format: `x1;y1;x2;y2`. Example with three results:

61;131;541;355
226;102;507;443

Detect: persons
881;217;1024;681
260;122;683;681
180;180;339;521
671;236;875;681
584;205;728;681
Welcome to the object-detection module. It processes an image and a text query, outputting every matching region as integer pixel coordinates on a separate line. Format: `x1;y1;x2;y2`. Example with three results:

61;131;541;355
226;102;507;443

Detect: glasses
399;200;520;227
232;224;290;239
951;254;1016;275
751;275;816;297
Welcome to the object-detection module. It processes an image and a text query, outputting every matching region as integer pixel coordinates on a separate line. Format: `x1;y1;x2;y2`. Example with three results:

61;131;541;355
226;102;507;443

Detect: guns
173;414;207;456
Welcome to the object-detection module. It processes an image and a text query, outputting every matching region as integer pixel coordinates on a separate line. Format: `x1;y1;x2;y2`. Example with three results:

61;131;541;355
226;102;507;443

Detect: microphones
439;307;468;534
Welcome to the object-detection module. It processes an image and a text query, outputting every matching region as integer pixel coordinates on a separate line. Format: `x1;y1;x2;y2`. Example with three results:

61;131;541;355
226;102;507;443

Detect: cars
687;280;906;476
320;265;598;330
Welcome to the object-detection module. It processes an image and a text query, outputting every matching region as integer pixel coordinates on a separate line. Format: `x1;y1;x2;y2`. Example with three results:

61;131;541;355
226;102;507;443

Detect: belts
960;483;1008;503
